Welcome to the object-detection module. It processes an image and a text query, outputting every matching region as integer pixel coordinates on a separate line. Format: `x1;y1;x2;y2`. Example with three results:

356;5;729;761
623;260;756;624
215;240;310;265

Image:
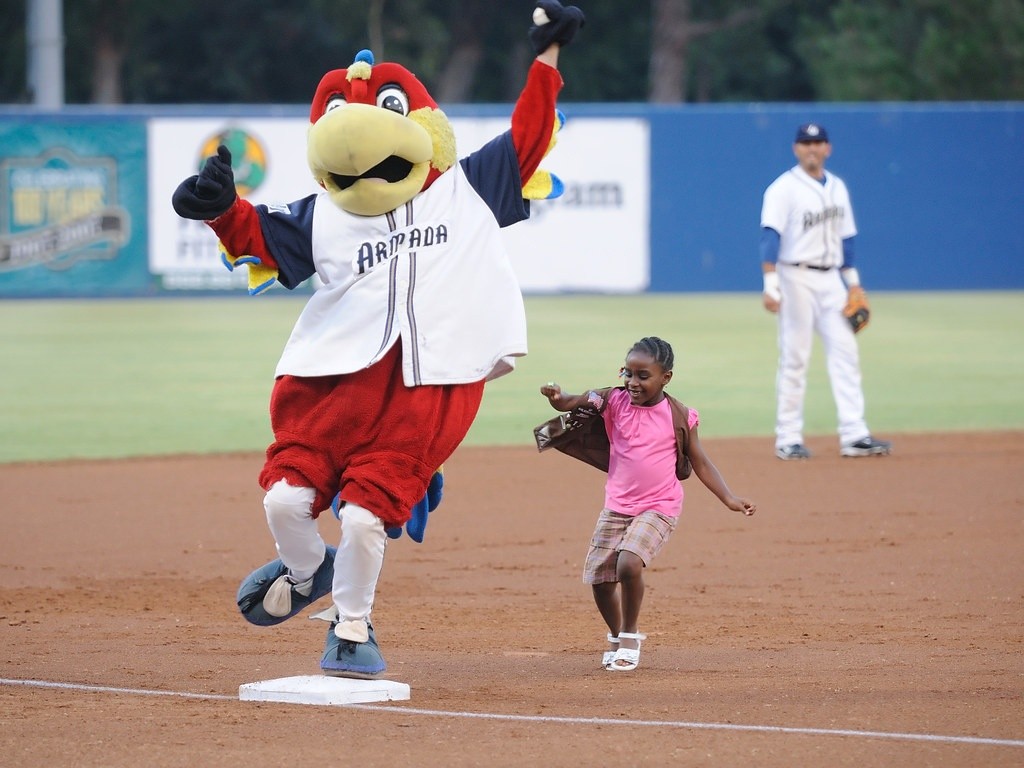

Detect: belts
792;264;830;271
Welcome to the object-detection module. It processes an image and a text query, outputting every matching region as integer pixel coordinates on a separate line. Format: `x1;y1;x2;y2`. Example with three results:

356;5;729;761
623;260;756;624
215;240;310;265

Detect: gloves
762;271;781;311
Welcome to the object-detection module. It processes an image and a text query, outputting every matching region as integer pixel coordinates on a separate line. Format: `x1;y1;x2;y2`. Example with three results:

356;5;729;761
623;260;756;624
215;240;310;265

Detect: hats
794;124;829;145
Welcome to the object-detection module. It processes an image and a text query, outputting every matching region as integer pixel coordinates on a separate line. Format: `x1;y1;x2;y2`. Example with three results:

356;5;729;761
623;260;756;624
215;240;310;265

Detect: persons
758;123;892;461
533;336;756;674
170;1;588;685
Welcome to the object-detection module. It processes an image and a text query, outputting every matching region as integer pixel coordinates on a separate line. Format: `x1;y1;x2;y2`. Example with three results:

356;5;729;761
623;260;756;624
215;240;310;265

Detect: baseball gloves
842;278;868;334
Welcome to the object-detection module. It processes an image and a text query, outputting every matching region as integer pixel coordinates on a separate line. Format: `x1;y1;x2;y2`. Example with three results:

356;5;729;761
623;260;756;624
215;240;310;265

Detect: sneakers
840;435;891;456
777;444;812;460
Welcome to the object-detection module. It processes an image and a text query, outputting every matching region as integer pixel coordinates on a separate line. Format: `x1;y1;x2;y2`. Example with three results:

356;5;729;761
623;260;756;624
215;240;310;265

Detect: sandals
611;630;646;671
602;633;620;670
